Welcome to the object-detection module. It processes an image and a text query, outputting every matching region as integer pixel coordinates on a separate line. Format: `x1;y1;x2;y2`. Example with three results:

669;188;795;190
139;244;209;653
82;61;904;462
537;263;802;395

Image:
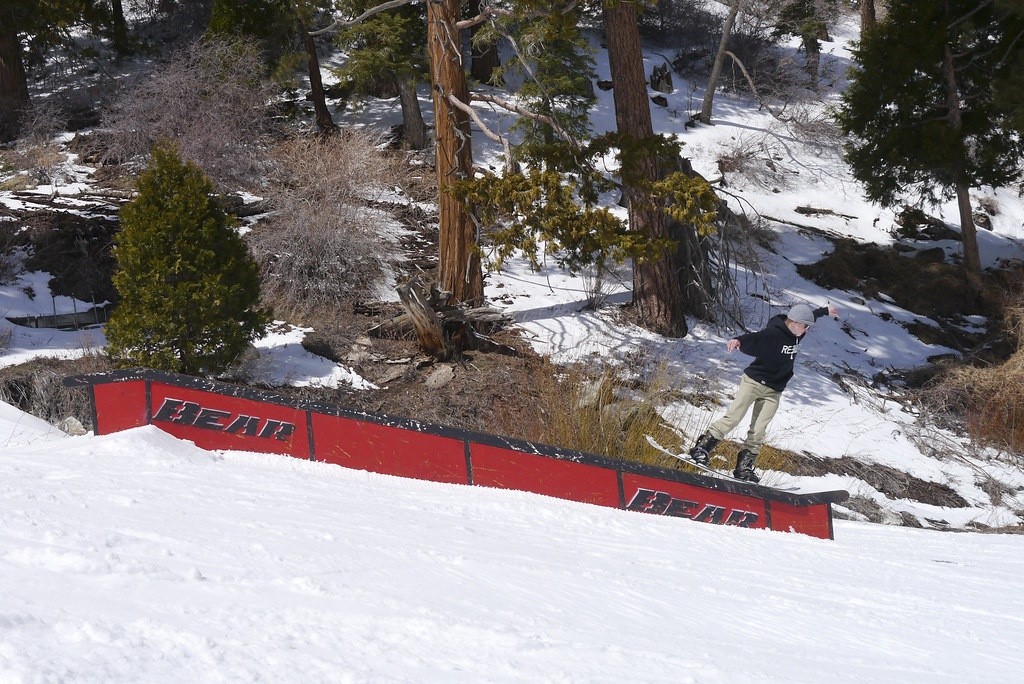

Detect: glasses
800;323;811;330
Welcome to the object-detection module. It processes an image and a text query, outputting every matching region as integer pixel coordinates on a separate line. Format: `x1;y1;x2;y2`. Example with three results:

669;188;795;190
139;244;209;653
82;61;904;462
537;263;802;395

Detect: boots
689;430;720;468
733;448;758;483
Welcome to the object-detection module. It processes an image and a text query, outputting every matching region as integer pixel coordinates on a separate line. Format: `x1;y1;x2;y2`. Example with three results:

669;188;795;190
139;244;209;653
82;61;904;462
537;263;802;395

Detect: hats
788;304;815;326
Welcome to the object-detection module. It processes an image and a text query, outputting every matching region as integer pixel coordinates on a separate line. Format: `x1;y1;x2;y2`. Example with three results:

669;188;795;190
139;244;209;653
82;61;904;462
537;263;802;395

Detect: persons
690;304;839;483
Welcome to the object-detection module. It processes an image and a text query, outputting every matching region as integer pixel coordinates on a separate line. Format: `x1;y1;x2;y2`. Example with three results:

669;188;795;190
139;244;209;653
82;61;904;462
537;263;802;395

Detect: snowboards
643;431;800;492
